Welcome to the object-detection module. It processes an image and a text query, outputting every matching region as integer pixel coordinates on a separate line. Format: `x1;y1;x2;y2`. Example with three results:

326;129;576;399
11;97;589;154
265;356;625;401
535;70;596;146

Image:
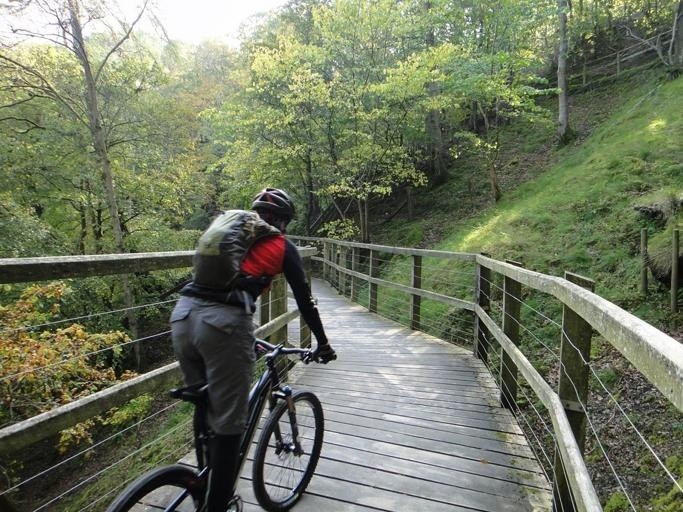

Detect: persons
170;187;336;512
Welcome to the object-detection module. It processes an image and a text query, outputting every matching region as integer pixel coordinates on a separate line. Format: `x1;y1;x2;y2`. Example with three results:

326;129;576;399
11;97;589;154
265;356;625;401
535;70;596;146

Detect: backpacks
191;210;282;293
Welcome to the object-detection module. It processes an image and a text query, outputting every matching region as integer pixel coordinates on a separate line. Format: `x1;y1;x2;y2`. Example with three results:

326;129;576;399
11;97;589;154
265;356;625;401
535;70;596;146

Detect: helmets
251;187;296;220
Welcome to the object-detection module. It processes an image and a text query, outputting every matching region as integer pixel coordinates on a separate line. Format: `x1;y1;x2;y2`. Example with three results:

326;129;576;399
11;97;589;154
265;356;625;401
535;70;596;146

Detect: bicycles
105;338;337;512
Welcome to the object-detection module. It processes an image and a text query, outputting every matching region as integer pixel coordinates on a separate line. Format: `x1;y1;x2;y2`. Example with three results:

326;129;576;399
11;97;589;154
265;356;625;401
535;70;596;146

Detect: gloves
312;343;337;364
